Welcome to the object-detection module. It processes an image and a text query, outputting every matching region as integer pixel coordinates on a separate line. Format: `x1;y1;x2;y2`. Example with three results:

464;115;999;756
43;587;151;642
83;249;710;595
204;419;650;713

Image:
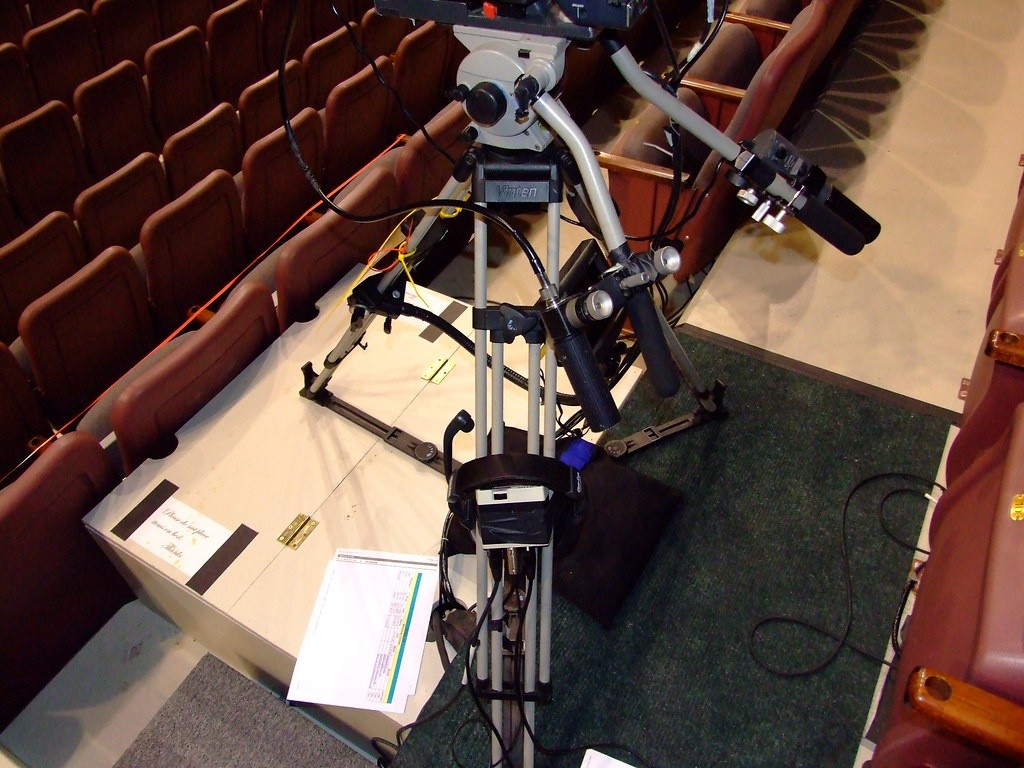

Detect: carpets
391;324;962;768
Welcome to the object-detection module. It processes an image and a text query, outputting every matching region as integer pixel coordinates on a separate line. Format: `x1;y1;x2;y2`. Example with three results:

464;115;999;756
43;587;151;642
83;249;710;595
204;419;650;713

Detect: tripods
298;25;878;768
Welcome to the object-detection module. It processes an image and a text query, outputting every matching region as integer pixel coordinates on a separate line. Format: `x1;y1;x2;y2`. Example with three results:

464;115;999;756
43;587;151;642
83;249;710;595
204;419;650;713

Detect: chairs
0;0;857;730
